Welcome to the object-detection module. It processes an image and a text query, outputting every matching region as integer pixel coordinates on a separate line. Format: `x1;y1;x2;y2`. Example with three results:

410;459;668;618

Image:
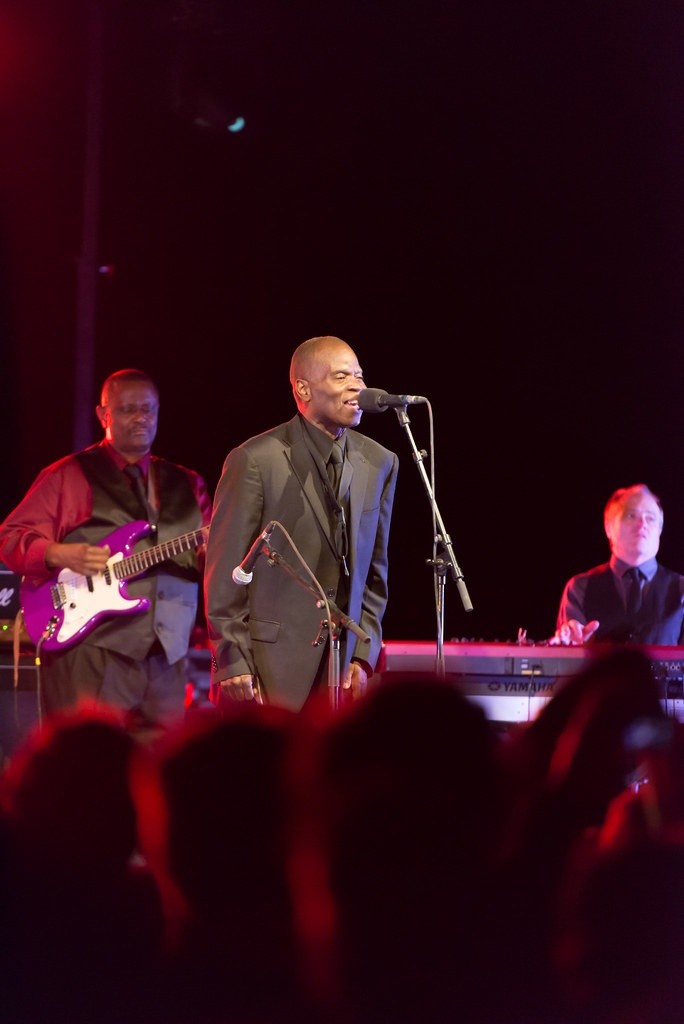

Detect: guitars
18;520;211;654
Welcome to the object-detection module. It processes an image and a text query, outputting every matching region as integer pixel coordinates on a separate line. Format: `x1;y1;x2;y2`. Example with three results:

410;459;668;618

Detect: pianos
378;636;684;686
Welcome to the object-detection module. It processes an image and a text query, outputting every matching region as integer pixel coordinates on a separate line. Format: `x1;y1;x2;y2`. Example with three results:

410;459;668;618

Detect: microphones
232;521;275;586
358;388;428;413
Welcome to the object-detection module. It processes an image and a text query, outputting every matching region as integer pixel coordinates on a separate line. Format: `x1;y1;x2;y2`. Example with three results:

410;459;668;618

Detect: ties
330;443;343;494
123;464;157;534
627;568;641;621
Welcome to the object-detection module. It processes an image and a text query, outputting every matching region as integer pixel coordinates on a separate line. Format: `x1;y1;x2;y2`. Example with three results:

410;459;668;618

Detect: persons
204;335;399;713
2;643;684;1023
545;484;684;646
0;368;214;721
